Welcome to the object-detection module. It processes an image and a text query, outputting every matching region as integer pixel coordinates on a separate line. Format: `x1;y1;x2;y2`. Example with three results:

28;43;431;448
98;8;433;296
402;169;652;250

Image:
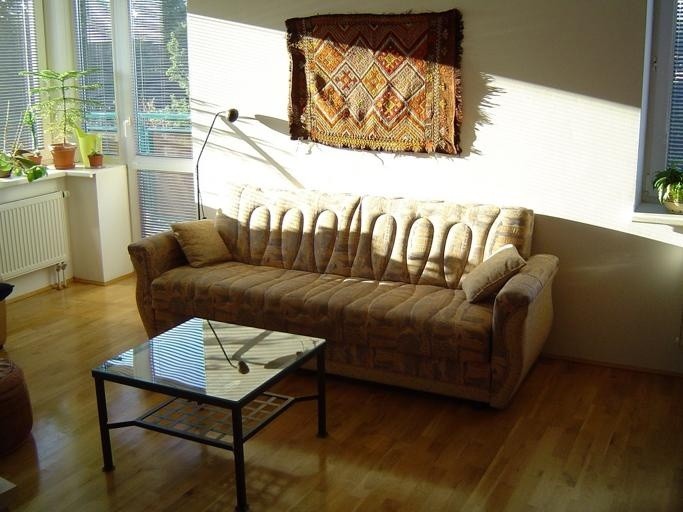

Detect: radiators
0;189;70;292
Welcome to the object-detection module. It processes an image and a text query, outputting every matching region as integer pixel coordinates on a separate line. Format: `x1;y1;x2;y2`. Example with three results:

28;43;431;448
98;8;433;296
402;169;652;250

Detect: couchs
127;184;559;409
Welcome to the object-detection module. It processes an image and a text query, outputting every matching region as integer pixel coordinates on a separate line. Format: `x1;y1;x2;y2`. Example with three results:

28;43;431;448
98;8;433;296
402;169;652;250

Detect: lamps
194;108;237;220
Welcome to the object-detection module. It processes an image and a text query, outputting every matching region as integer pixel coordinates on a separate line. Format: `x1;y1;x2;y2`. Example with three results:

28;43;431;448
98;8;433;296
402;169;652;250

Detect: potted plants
0;149;48;182
649;162;682;214
88;149;103;166
19;69;102;169
21;110;42;164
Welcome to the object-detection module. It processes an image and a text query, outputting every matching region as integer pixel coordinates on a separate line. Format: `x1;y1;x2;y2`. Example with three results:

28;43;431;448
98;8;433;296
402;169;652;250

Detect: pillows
460;243;527;301
167;218;231;268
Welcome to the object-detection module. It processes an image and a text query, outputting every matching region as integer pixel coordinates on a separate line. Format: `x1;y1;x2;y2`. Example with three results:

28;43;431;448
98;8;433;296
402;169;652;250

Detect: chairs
0;279;33;456
